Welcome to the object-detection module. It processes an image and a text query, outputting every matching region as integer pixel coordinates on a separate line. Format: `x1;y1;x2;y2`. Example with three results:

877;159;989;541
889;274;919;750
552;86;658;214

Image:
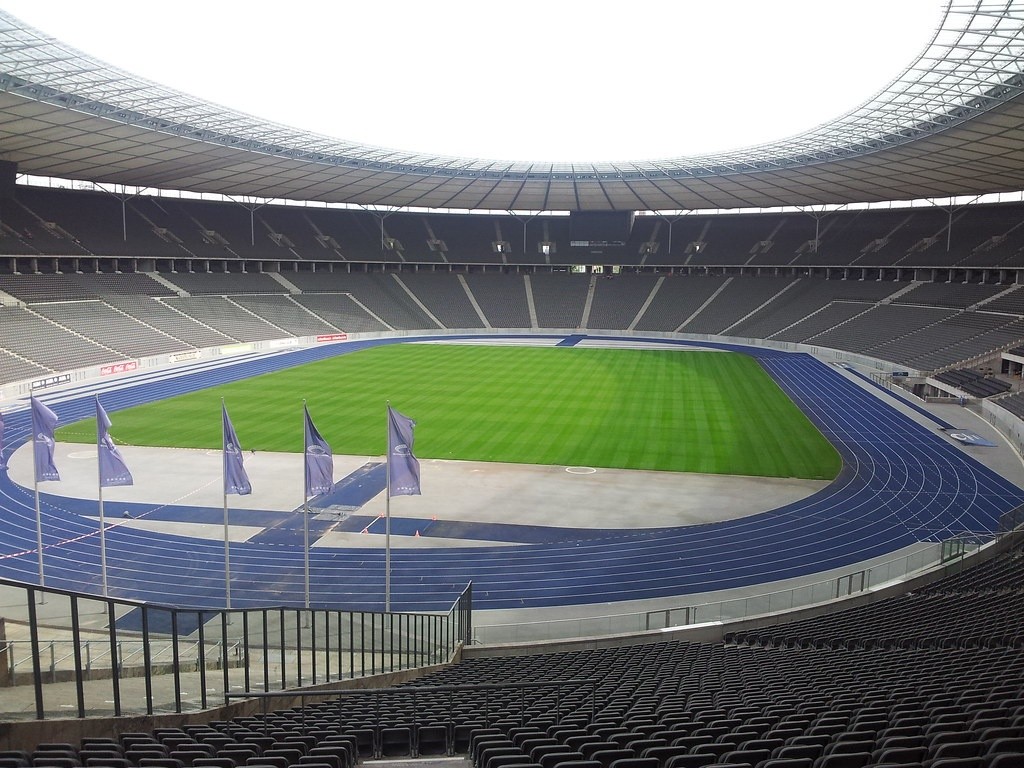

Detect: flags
0;413;9;470
96;400;133;488
31;396;61;483
389;407;421;496
223;406;252;495
304;406;336;497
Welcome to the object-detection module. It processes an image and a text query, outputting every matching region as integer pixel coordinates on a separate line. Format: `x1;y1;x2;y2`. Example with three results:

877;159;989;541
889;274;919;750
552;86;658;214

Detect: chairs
0;533;1024;768
